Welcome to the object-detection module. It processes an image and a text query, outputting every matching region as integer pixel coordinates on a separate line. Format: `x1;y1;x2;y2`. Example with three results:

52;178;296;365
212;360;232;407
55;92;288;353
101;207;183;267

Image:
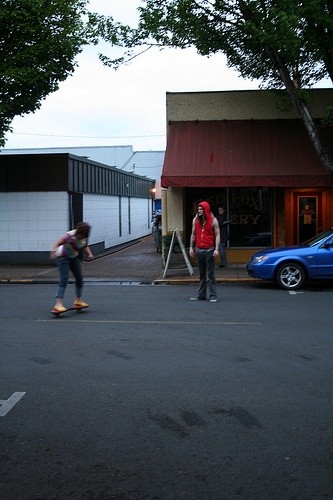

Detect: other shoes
219;265;225;268
75;301;87;306
55;306;66;312
210;299;217;302
190;297;199;301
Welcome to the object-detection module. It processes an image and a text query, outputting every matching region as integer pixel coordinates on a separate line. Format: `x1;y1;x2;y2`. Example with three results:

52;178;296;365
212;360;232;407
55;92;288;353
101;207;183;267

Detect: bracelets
215;249;219;251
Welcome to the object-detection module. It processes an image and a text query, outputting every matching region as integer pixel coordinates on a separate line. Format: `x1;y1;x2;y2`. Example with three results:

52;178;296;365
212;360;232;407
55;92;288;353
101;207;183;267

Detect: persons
50;222;94;313
216;206;229;268
190;200;221;303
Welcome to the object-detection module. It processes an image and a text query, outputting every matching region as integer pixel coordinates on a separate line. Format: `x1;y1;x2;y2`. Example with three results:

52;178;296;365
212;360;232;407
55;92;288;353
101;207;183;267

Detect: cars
246;228;333;290
